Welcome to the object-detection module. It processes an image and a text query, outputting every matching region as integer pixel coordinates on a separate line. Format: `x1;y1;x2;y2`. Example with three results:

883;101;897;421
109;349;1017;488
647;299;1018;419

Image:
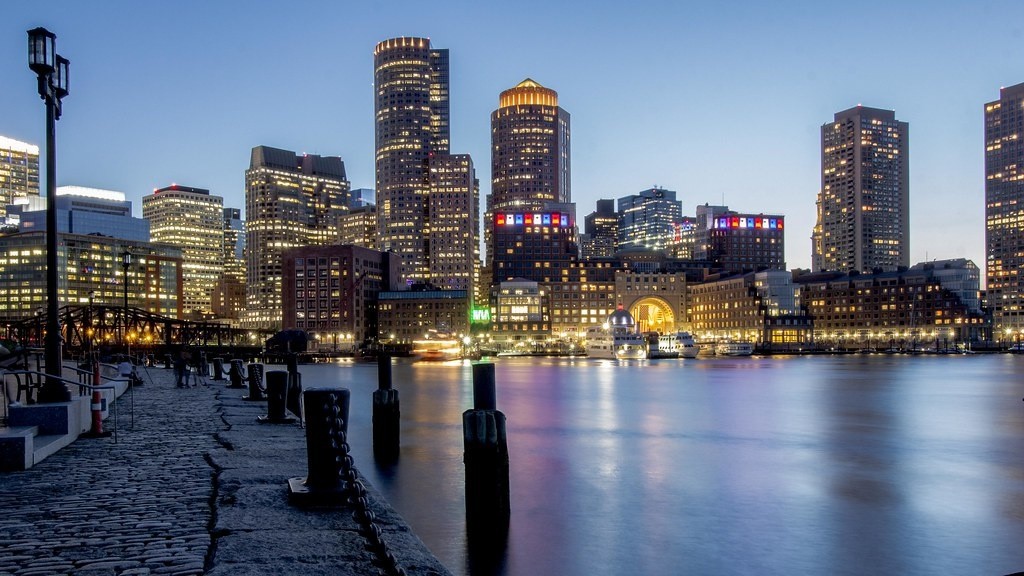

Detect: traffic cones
77;361;112;439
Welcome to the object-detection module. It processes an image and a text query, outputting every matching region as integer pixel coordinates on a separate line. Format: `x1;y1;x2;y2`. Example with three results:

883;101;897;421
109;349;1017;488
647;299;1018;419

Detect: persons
120;357;144;386
168;344;208;389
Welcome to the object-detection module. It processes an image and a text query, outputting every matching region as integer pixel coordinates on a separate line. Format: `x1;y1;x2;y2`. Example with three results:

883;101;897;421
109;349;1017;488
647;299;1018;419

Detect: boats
409;326;465;362
715;341;753;357
658;331;701;360
497;348;528;359
584;323;648;362
650;350;678;360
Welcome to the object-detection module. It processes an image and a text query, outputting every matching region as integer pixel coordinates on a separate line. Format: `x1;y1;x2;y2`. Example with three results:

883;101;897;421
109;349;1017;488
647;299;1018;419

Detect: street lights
122;249;131;357
86;292;95;357
26;25;69;405
